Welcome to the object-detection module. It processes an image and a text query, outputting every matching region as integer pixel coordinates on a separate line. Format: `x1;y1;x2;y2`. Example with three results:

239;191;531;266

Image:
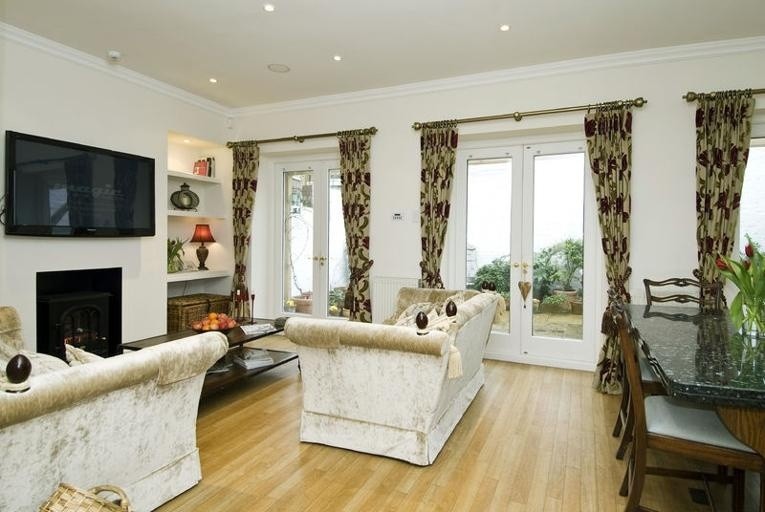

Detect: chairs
615;315;765;512
643;278;722;308
612;356;667;460
642;305;722;322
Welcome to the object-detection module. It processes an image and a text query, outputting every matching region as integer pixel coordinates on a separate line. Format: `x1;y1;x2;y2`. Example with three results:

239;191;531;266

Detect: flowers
709;233;765;334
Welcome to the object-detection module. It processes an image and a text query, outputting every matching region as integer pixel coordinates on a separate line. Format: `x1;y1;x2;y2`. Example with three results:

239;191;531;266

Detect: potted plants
285;212;312;314
548;235;583;312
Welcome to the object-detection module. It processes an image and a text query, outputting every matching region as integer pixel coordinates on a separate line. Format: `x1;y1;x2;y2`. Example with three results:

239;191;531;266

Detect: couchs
283;288;500;467
1;305;229;510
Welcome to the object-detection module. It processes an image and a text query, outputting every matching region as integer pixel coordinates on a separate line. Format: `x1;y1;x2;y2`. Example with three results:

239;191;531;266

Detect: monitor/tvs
6;130;156;238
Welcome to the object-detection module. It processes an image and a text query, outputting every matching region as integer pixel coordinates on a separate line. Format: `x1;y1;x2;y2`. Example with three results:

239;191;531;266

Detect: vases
743;301;765;336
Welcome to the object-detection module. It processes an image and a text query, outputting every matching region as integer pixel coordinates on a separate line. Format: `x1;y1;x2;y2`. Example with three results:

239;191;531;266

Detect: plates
184;321;244;335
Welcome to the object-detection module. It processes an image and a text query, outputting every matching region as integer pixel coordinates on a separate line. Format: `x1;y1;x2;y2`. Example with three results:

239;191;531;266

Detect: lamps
188;224;216;270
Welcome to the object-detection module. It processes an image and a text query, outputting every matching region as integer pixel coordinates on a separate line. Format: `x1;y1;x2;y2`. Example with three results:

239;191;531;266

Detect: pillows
439;292;465;316
393;314;416;327
64;343;104;367
396;302;432;323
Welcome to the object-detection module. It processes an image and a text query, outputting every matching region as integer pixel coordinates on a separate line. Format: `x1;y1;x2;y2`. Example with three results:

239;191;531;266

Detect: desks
622;303;765;459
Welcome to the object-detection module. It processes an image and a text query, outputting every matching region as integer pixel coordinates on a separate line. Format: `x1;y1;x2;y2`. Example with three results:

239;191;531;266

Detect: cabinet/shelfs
167;130;236;285
